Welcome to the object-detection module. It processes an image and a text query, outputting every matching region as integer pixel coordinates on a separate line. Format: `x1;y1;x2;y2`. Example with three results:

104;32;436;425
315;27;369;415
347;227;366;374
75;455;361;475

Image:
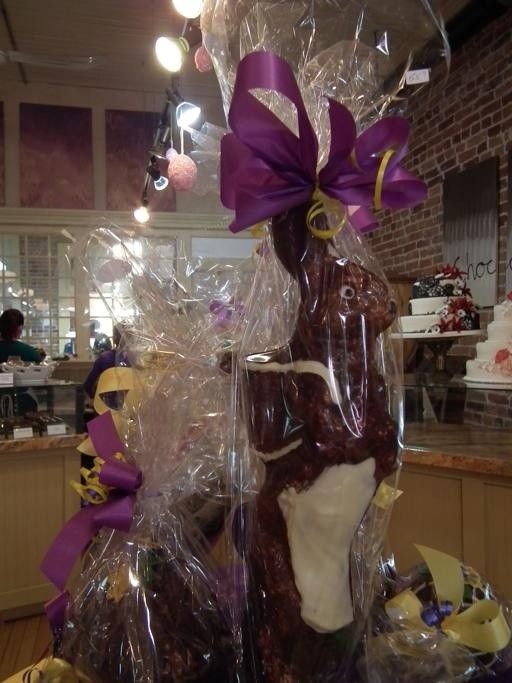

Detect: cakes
465;296;512;382
397;264;481;333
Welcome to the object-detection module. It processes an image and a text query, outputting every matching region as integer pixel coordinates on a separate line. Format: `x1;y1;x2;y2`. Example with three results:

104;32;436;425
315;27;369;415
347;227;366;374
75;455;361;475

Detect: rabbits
238;199;406;682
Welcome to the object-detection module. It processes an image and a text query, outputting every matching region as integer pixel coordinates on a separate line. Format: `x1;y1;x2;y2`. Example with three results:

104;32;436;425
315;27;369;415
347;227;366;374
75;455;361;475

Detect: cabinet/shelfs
385;464;510;612
0;454;62;620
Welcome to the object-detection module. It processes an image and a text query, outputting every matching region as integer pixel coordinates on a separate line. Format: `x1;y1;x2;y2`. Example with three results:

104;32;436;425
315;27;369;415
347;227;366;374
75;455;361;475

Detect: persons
1;309;46;416
81;319;136;412
88;320;112;352
